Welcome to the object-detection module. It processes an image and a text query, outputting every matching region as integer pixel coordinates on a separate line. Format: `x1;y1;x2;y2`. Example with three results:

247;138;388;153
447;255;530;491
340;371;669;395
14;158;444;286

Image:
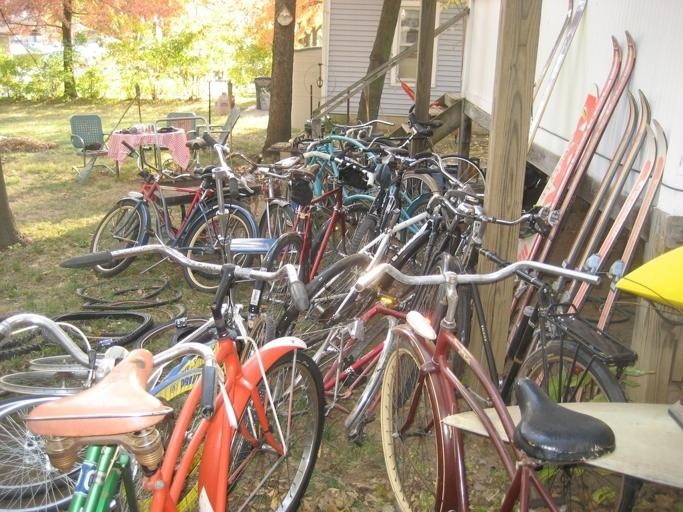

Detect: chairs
70;115;109;169
167;113;196;175
195;105;241;168
157;118;213;183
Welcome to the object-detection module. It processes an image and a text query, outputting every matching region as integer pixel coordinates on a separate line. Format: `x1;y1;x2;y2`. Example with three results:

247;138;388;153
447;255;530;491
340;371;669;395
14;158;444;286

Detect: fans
304;62;337;132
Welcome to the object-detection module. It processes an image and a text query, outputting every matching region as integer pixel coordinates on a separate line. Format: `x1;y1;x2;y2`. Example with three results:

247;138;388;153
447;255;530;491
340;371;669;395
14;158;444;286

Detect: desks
143;177;262;229
110;126;190;176
442;404;683;511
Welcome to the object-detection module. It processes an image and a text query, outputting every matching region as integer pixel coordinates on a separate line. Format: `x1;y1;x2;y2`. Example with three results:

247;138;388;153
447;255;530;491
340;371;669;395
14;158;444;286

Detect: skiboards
501;1;667;400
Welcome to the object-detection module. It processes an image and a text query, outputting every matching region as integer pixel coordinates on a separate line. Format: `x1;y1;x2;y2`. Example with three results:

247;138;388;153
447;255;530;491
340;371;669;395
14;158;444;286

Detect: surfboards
438;400;682;488
615;246;682;310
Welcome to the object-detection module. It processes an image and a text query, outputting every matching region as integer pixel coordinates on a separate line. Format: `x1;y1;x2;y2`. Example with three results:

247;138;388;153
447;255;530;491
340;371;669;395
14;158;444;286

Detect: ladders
312;6;470;121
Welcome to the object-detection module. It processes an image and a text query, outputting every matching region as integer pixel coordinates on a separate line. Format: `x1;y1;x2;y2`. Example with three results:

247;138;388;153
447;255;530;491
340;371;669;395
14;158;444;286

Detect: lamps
274;3;293;27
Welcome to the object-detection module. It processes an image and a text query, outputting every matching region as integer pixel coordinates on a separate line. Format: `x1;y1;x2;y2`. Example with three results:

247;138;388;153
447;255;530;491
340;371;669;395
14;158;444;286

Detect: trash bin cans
255;77;272;110
